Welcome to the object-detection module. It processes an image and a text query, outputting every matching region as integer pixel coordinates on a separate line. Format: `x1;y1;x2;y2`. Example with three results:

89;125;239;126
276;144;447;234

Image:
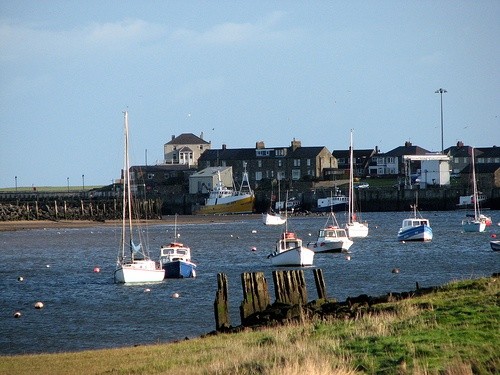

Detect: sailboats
261;170;287;226
462;147;500;251
113;110;165;285
341;129;369;238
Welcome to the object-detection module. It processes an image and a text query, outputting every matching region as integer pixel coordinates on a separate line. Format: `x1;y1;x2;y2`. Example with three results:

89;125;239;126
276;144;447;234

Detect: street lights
82;175;84;191
434;88;448;155
15;176;18;194
67;177;69;193
145;149;147;166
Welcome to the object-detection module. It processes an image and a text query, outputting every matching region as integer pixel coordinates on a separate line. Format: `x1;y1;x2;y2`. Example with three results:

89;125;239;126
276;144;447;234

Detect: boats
194;162;256;215
267;190;315;267
456;190;488;209
307;190;354;253
157;213;197;279
397;204;433;242
